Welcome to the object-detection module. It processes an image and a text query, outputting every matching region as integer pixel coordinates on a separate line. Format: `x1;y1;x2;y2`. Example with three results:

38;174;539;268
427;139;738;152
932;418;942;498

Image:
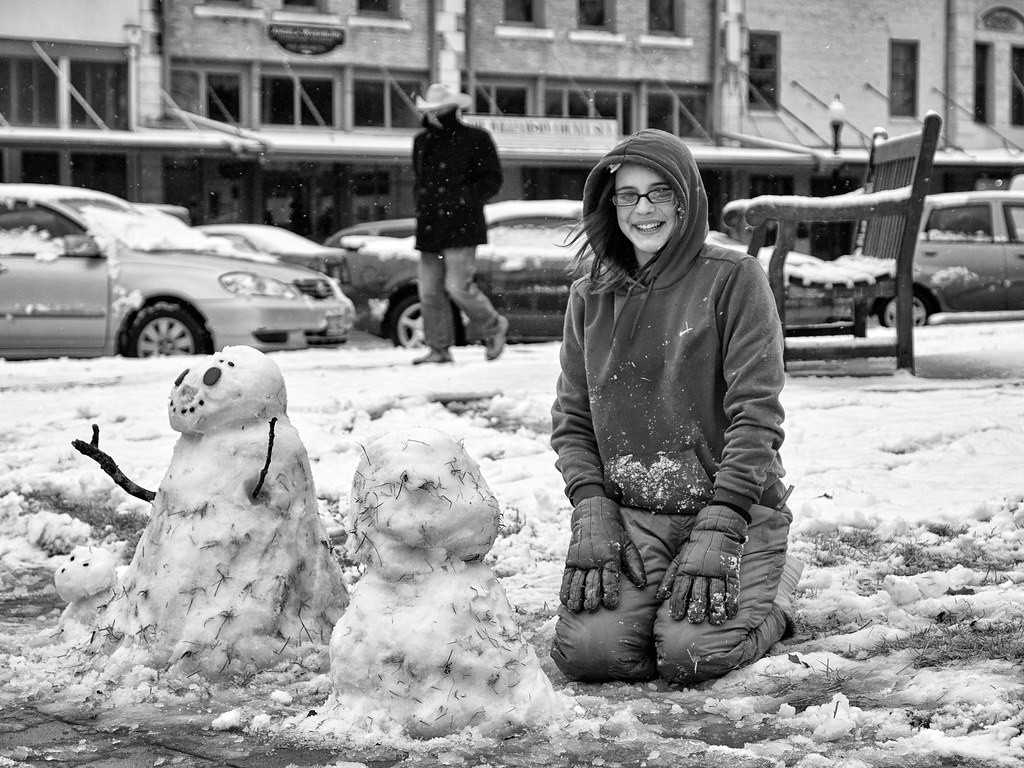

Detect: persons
548;128;806;683
411;81;509;364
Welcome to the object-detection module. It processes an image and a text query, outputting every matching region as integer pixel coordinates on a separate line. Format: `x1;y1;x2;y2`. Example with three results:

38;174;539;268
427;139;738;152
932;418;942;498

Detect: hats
416;81;474;111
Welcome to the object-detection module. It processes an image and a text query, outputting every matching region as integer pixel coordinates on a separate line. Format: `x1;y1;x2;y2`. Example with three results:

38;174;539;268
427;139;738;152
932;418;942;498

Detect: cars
194;221;354;297
873;189;1024;332
0;184;357;359
315;198;865;351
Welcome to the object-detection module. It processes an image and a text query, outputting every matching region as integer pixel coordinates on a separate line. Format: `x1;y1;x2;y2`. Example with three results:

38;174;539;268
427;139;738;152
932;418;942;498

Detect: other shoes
773;554;803;610
483;316;509;358
413;348;456;363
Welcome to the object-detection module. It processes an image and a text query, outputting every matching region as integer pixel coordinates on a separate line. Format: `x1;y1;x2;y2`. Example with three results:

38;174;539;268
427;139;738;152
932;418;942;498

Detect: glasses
612;187;676;206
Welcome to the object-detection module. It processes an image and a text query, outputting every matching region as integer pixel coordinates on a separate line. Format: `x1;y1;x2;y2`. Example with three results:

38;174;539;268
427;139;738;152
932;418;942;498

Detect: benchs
722;109;944;375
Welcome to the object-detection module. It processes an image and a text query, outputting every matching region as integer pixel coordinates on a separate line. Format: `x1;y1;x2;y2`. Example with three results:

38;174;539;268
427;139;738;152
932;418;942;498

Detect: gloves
655;505;749;625
559;496;646;612
